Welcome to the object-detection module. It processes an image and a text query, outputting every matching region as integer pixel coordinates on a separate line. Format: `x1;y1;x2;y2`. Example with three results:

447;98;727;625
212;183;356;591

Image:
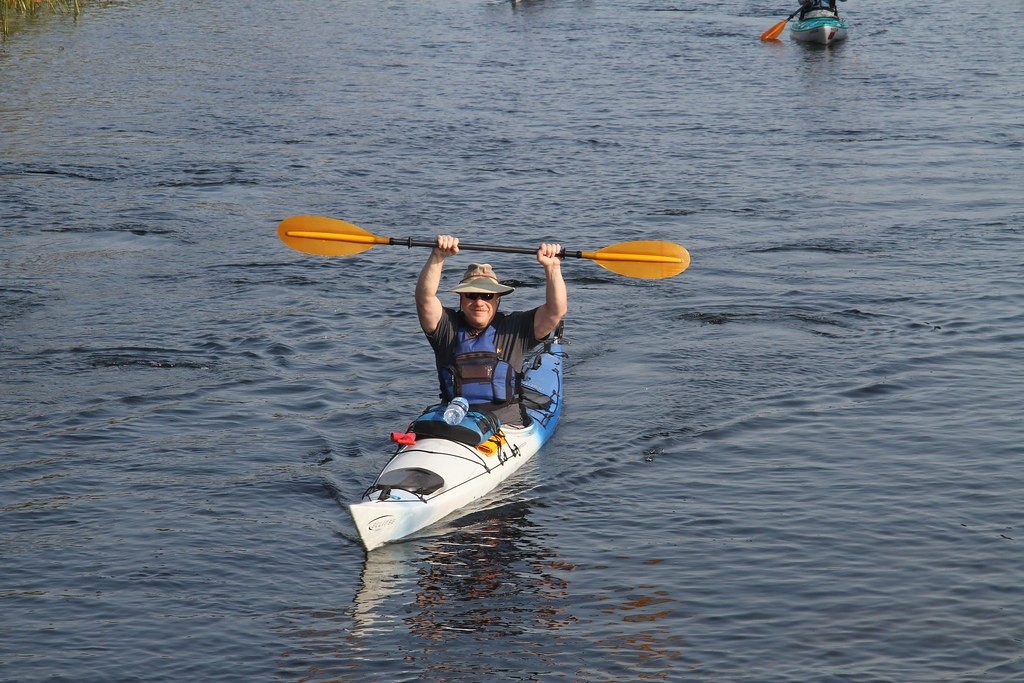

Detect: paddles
276;213;690;278
761;7;802;41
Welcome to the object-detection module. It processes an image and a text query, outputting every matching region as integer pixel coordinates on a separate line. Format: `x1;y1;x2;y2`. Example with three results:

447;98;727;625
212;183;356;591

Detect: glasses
464;291;496;300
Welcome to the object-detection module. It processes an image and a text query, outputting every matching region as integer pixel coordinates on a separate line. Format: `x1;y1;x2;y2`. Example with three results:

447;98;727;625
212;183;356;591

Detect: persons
414;235;568;426
798;0;847;8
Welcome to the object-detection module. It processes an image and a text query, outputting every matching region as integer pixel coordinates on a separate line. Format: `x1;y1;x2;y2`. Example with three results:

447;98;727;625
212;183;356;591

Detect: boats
790;15;848;43
347;324;564;552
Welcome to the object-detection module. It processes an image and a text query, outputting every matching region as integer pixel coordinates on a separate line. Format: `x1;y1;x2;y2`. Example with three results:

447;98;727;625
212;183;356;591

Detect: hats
452;262;515;296
